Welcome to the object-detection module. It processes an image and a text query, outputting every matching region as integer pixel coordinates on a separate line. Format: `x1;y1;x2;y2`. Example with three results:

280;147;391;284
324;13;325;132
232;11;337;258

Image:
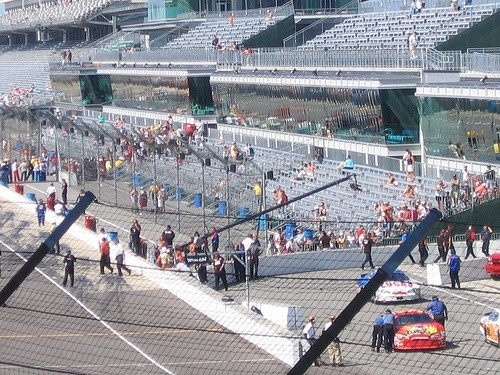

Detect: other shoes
371;348;375;351
389;350;392;353
331;362;335;367
376;346;379;352
385;349;388;352
338;363;344;367
320;362;326;365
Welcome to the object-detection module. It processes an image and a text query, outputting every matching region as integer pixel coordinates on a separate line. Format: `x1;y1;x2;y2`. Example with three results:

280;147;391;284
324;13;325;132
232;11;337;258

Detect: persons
426;296;448;326
409;32;418;59
449;131;500;160
302;317;324;366
465;225;476;258
447;250;461;288
0;134;57;184
0;83;35;108
169;103;359;140
227;14;233;23
410;0;422;14
481;225;492;257
322;316;344;366
419;235;428;267
219;141;500;270
63;50;72;65
73;115;205;184
371;309;397;353
212;36;252;54
266;10;272;20
34;179;263;294
434;224;456;262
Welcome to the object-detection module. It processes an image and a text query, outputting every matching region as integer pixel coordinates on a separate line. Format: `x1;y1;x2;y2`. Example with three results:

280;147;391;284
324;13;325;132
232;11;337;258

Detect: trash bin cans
27;193;35;199
259;214;269;232
133;175;141;186
195;193;202;209
1;179;7;184
239;207;249;219
175;188;183;200
218;201;228;216
286;222;296;239
108;231;118;240
65;209;72;216
303;230;313;241
164;183;171;197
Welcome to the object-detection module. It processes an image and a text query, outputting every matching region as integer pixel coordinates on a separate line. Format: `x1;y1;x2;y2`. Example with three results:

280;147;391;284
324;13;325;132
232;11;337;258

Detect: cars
478;307;500;350
388;309;447;352
357;268;421;305
484;245;500;280
